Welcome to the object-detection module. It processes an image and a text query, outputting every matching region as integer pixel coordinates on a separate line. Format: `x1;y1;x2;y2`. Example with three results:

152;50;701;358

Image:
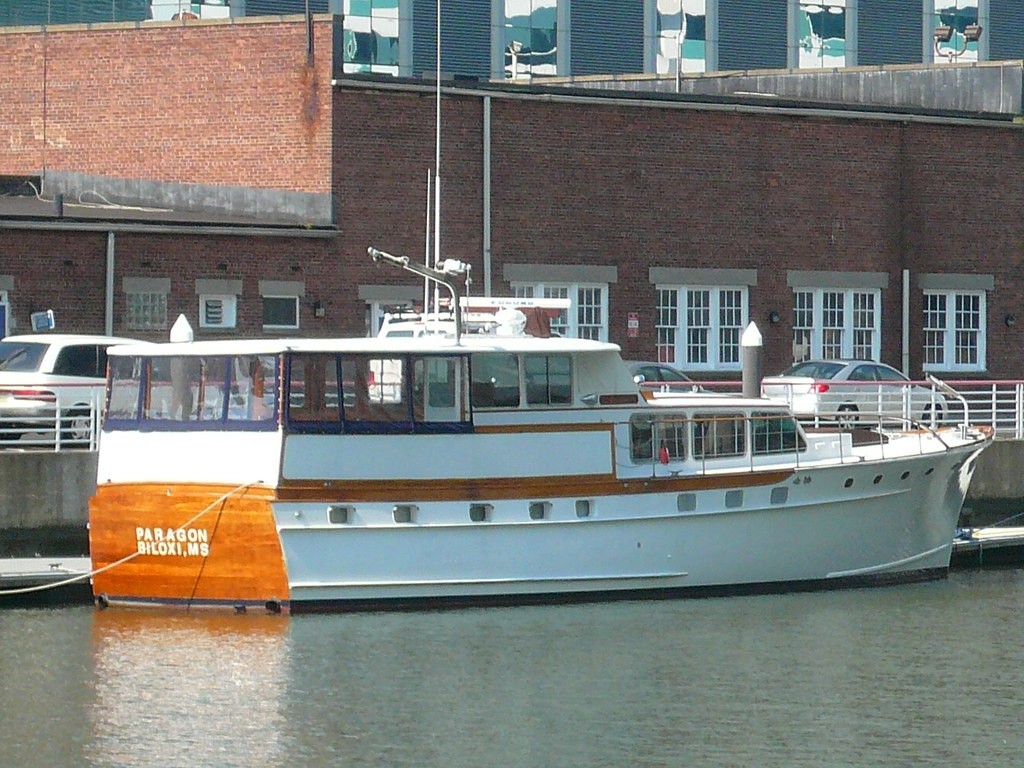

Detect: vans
366;318;536;395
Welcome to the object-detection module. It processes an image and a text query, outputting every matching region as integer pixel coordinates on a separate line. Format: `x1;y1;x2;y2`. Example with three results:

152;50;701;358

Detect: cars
762;357;950;427
620;360;746;431
0;334;215;447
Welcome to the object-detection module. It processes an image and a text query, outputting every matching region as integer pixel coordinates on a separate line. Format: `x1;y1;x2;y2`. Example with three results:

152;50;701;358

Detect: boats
85;244;998;610
